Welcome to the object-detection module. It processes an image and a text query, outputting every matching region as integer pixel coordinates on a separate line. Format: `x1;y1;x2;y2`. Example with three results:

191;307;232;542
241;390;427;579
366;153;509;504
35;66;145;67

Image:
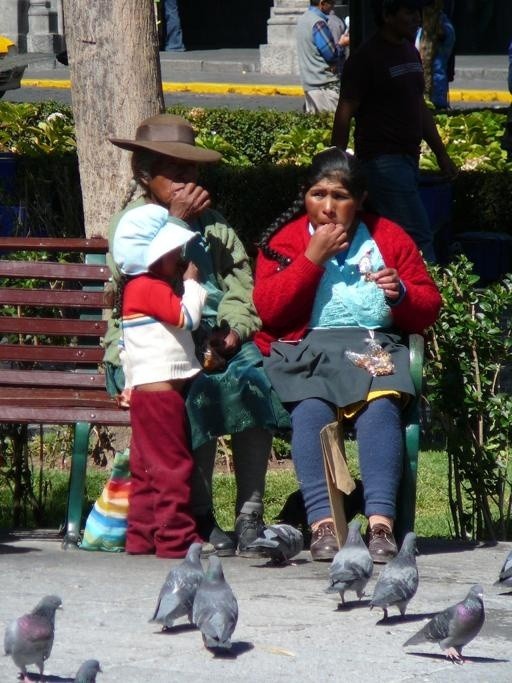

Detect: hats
108;114;222;162
113;203;202;275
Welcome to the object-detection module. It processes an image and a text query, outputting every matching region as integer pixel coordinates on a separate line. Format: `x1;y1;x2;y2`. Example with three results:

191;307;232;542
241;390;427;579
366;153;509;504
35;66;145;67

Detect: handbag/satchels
79;448;131;550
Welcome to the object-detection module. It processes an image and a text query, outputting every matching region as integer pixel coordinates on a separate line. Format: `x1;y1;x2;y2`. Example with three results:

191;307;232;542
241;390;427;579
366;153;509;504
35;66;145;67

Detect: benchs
1;235;431;538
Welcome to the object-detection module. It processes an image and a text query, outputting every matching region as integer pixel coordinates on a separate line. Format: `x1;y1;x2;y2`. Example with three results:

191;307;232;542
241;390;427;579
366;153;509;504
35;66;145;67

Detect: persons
111;204;219;560
250;147;441;563
294;0;455;113
328;3;462;269
101;111;291;560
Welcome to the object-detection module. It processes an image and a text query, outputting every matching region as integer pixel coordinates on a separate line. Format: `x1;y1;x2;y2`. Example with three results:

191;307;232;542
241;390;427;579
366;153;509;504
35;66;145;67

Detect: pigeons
491;547;512;591
365;530;422;623
244;522;307;568
189;553;242;653
400;582;486;668
2;592;67;683
318;518;376;611
147;539;207;633
69;655;105;683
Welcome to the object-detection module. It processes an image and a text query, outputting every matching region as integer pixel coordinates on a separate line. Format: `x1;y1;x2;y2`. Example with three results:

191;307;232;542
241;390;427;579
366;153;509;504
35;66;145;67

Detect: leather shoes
200;512;398;563
